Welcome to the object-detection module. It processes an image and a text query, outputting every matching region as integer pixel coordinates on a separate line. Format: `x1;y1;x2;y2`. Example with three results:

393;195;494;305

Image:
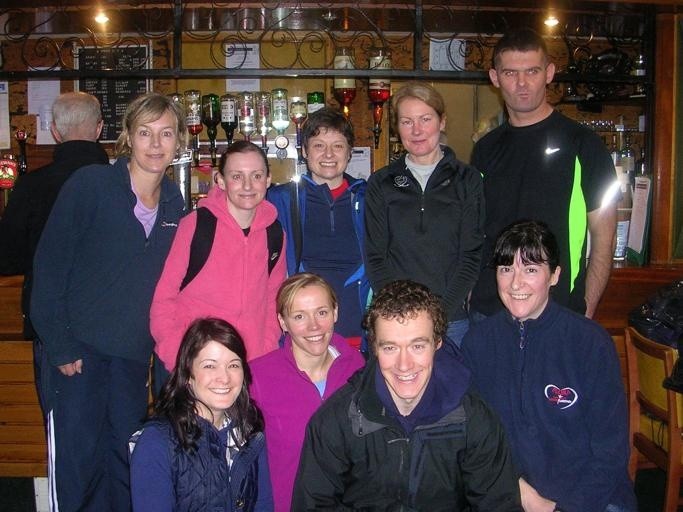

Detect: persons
265;108;374;360
365;84;486;347
471;26;621;320
244;273;366;510
459;218;640;512
130;318;275;511
0;92;109;437
289;283;521;510
31;94;189;512
149;140;287;374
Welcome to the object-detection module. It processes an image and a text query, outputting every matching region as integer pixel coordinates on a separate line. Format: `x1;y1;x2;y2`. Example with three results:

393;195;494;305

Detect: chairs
624;325;683;511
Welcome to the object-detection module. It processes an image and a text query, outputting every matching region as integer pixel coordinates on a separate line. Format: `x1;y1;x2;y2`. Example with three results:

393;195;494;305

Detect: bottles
610;134;647;269
168;88;326;165
183;8;306;30
631;54;645;92
333;45;390;123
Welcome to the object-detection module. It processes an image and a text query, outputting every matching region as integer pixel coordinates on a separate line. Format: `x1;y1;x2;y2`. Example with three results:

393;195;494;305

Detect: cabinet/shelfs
1;0;683;277
